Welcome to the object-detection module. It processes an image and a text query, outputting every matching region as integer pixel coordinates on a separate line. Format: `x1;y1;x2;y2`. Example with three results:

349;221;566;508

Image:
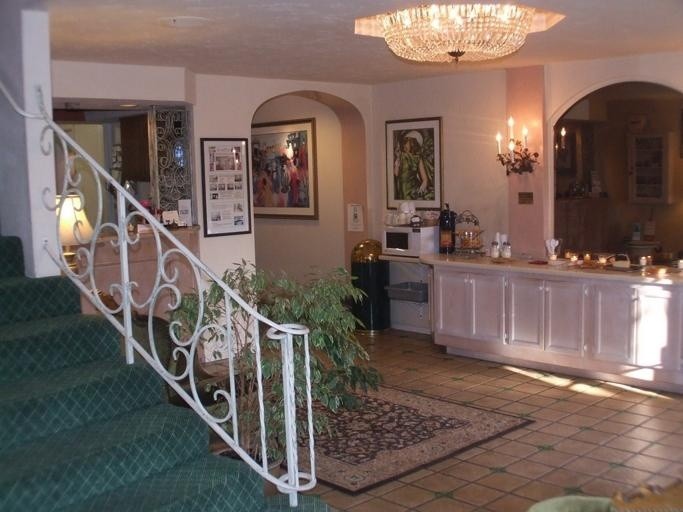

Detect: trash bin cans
351;239;390;336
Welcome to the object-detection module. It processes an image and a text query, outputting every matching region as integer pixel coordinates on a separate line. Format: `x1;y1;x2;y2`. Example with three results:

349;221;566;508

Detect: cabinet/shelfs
627;129;680;206
430;263;504;346
592;285;682;373
504;272;590;357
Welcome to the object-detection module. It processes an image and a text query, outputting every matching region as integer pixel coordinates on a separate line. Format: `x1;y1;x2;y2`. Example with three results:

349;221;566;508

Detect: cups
548;247;608;265
647;255;654;265
640;256;647;266
678;259;683;270
383;213;411;225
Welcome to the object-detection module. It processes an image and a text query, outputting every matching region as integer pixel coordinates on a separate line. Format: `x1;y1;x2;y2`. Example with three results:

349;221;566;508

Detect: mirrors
551;81;683;265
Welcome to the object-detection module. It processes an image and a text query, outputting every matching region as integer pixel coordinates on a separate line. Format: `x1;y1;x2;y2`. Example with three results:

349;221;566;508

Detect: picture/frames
200;137;255;237
251;116;319;220
384;116;443;211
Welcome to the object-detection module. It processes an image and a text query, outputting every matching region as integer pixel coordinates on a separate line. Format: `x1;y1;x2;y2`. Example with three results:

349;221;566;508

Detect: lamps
353;3;566;63
55;193;96;273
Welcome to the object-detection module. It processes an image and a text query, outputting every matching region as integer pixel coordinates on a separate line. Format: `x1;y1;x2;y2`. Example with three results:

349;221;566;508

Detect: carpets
240;376;534;496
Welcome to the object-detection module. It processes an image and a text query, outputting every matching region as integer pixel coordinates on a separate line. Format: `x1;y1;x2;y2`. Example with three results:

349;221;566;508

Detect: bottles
137;200;153;224
439;203;511;259
656;242;672;265
631;216;640;241
643;207;657;241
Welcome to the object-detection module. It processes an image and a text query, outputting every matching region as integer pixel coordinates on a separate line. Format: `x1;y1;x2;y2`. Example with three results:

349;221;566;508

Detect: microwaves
381;226;439;257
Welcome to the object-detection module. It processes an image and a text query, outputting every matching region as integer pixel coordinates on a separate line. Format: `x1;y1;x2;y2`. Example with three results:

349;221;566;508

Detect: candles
494;113;540;177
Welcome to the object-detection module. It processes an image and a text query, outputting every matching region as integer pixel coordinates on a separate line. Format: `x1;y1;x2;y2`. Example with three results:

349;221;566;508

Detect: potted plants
165;264;384;496
